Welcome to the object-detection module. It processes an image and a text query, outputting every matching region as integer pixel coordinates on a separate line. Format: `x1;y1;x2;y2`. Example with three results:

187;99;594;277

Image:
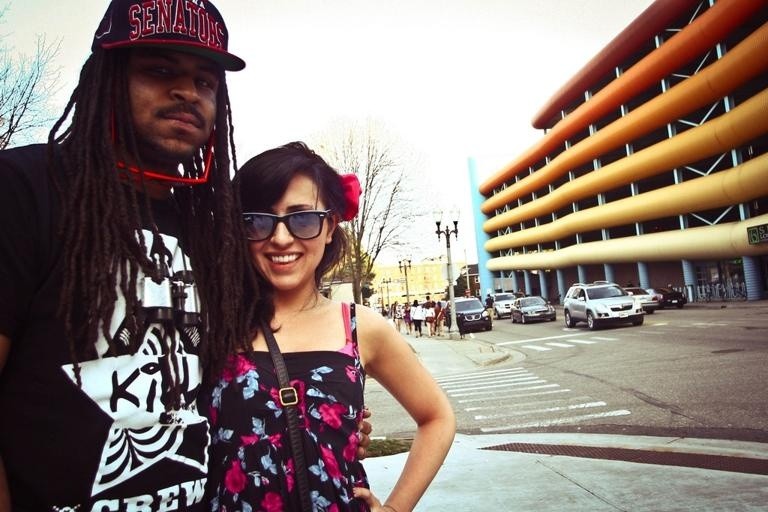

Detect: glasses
108;78;216;200
240;209;332;241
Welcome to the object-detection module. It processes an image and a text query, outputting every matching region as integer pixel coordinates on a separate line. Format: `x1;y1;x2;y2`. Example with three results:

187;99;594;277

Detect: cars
433;280;688;331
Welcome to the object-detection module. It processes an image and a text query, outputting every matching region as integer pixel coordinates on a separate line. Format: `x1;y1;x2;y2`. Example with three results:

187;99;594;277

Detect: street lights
383;278;394;305
398;259;415;306
435;209;465;340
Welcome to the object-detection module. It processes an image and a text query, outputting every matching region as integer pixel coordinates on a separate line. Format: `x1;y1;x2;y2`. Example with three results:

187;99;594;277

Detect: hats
92;0;245;71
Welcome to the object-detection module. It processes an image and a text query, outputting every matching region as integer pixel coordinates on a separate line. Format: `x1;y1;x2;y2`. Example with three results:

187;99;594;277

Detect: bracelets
380;500;397;512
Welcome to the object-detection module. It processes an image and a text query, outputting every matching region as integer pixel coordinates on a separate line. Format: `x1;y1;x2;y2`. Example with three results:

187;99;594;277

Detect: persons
208;142;460;512
360;291;453;340
484;293;494;328
715;280;726;302
0;0;375;511
625;279;632;286
704;282;712;304
667;281;673;290
